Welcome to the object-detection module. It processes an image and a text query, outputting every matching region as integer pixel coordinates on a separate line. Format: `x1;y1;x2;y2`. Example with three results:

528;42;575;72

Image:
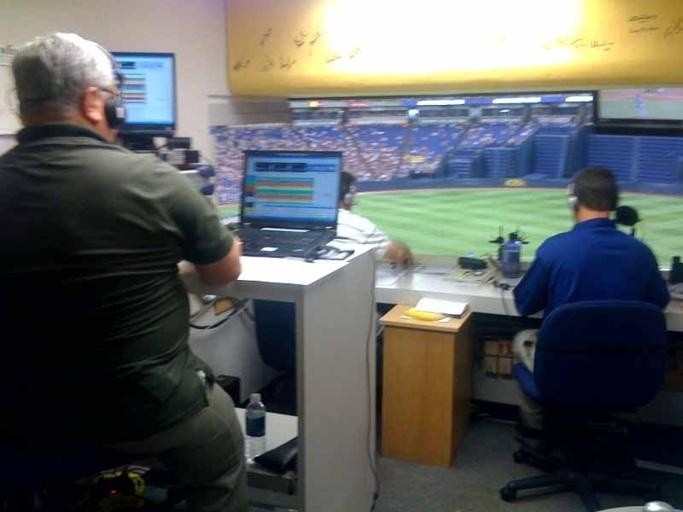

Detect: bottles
243;394;268;460
501;233;521;278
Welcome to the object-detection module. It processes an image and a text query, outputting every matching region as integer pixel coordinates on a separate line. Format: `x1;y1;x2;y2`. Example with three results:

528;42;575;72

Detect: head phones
95;43;126;125
343;182;359;206
564;168;577;213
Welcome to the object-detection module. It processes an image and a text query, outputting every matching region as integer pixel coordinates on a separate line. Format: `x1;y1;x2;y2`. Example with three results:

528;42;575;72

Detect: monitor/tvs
108;51;176;151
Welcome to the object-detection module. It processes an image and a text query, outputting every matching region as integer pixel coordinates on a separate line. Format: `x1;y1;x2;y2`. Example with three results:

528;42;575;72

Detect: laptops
222;149;344;258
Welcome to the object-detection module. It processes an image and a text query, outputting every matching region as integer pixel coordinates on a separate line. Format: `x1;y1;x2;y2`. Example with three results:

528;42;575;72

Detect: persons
1;30;253;512
504;167;672;460
331;174;414;269
213;95;572;203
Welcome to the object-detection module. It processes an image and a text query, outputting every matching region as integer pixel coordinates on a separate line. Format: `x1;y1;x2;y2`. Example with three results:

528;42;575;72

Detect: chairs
499;303;665;512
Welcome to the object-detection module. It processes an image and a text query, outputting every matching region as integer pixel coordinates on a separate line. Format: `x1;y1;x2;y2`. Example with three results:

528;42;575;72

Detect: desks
171;228;380;510
369;247;682;431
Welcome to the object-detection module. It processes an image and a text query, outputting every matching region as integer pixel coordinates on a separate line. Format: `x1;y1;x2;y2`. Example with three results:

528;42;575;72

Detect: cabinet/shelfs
380;304;473;469
